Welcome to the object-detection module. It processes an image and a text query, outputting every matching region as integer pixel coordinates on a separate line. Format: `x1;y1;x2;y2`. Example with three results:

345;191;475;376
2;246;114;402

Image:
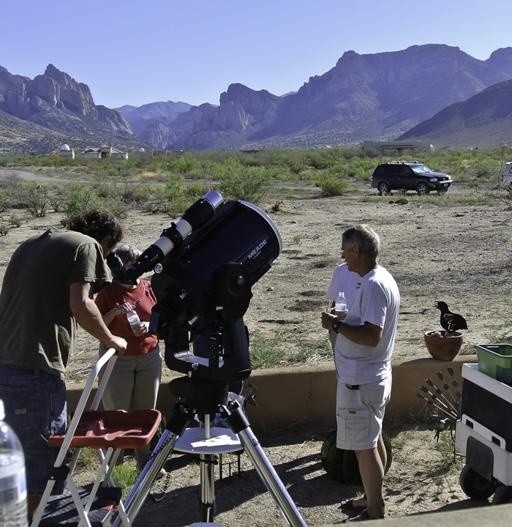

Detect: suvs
370;159;454;196
499;162;512;197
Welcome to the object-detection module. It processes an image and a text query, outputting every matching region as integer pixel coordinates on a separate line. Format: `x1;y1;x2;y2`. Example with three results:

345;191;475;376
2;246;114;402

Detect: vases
421;328;464;361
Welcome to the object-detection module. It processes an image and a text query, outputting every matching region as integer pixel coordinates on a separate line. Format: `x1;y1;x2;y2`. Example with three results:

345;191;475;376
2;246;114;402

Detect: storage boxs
459;359;511;451
473;340;511;382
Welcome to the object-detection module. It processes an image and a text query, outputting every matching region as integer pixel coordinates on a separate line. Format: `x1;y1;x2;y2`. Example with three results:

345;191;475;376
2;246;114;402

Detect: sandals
340;493;386;523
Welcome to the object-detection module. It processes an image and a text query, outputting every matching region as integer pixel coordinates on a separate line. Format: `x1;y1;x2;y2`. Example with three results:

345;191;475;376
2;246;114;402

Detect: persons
318;224;401;523
94;246;168;479
325;262;362;357
0;207;128;520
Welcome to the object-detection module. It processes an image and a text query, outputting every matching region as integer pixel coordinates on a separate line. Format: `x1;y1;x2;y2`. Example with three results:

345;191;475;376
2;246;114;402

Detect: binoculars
108;191;309;375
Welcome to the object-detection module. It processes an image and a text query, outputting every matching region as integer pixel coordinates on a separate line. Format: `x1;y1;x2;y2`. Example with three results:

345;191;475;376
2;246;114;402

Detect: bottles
0;396;31;527
334;291;346;311
127;309;143;336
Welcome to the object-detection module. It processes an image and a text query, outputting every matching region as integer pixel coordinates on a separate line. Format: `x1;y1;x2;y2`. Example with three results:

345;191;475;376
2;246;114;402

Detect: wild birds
431;301;468;332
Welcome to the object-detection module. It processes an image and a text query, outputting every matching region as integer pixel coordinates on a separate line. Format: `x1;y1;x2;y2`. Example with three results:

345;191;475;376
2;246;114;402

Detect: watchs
332;321;343;334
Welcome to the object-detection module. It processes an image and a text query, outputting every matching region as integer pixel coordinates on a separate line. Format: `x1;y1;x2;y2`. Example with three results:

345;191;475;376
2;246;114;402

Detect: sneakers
137;461;167;479
